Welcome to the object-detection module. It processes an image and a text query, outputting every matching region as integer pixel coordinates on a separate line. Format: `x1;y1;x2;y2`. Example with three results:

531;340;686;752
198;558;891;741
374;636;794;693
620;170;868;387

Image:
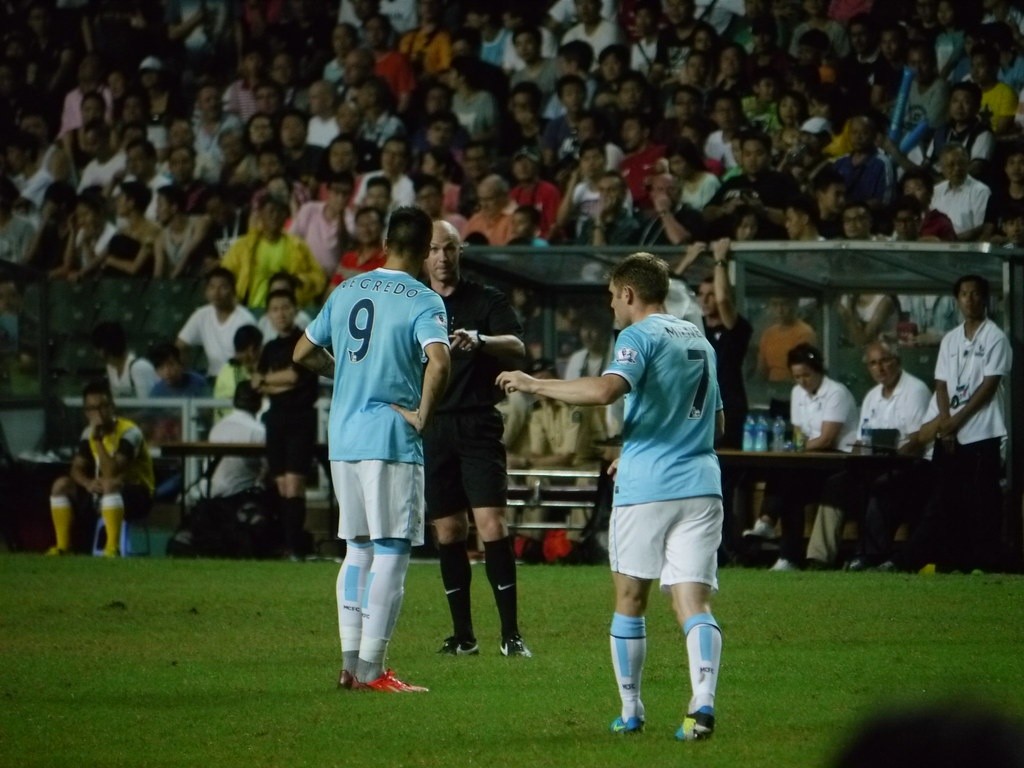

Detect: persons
881;389;941;568
291;205;451;694
493;287;623;568
251;289;321;560
491;0;1024;379
914;275;1015;573
806;339;935;567
668;235;751;563
417;221;532;658
0;0;516;562
46;381;156;556
741;342;859;570
494;252;731;743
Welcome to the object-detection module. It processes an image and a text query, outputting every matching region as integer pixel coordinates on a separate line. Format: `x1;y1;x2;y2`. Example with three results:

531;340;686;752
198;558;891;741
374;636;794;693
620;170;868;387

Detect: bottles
742;415;755;452
754;416;769;452
861;418;872;447
772;416;786;452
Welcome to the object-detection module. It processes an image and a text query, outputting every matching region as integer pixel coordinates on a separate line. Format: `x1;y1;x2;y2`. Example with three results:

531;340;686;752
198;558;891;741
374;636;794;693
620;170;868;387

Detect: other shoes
48;545;77;558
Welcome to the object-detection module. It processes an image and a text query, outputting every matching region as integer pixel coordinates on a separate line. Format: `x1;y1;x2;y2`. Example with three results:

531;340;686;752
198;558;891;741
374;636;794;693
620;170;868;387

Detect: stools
93;515;149;557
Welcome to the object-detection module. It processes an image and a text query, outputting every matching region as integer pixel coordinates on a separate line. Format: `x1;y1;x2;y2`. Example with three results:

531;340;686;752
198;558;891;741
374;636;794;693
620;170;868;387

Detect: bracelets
715;259;729;267
259;374;267;385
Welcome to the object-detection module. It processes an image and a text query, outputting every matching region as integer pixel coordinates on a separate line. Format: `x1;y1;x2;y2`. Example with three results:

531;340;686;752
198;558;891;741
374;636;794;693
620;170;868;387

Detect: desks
717;449;864;570
149;442;274;507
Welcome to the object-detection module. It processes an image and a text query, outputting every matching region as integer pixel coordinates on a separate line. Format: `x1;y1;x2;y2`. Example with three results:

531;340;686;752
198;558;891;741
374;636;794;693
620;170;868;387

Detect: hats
513;144;541;165
798;114;834;141
138;56;164;71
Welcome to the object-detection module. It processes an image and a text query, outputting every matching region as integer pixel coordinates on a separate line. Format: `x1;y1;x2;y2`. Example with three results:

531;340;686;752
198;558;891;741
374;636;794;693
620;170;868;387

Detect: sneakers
743;520;777;544
610;713;646;734
339;670;354;689
674;702;714;741
769;556;801;573
436;634;480;656
501;638;533;660
351;661;429;694
